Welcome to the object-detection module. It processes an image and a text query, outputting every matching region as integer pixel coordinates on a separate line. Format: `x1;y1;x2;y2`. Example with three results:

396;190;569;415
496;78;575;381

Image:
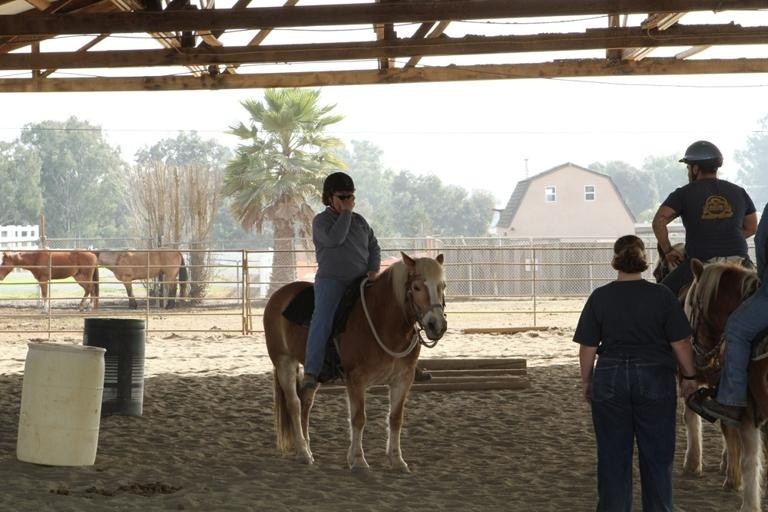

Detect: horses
0;248;100;313
88;248;187;310
261;249;449;474
650;240;768;512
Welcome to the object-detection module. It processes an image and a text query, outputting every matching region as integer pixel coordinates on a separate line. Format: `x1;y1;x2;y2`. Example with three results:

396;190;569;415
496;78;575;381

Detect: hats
614;236;646;256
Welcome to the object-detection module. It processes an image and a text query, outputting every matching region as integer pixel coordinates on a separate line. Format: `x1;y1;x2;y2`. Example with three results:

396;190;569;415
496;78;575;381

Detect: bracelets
663;248;672;255
683;374;697;380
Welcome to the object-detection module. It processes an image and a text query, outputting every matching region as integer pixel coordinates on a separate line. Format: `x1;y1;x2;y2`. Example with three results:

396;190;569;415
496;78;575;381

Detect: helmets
323;172;354;193
678;141;723;165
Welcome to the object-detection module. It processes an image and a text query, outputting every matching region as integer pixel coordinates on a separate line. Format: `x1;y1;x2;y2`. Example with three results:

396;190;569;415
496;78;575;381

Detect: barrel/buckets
83;315;144;418
16;341;105;467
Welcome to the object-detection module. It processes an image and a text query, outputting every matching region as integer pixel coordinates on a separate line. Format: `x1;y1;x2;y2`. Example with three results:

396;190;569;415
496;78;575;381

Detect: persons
700;203;767;428
651;141;757;298
573;234;699;512
301;172;431;391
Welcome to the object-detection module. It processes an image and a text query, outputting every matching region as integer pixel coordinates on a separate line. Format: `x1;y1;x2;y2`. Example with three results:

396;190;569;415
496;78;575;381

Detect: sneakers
301;374;317;389
414;366;432;382
701;397;746;428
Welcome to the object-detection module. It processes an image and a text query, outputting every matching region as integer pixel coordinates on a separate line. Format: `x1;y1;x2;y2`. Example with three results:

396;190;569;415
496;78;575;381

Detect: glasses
333;194;353;200
